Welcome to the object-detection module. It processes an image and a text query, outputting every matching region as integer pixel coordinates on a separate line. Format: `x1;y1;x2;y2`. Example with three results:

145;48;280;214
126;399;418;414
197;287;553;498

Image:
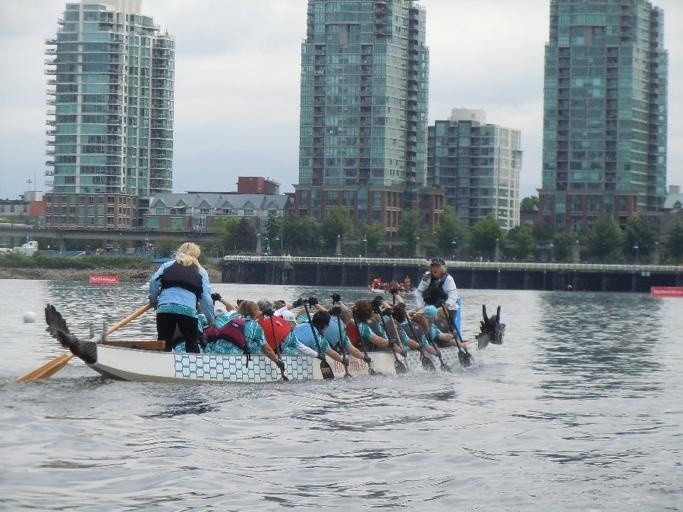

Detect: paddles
352;310;385;376
379;311;407;376
389;314;411;375
336;312;352;378
440;303;470;367
449;313;471;358
15;303;153;382
427;332;451;372
406;312;435;372
270;315;288;381
303;304;333;379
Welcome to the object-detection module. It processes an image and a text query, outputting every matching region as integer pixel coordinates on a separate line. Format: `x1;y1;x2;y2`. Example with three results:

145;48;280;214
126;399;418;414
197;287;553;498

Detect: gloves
293;299;306;306
331;293;341;302
398;302;406;307
276;359;284;368
400;351;407;355
319;352;324;359
263;309;273;315
363;355;371;362
309;297;319;304
416;344;421;349
391;288;397;294
342;359;350;365
237;299;243;306
374;306;380;314
211;294;221;300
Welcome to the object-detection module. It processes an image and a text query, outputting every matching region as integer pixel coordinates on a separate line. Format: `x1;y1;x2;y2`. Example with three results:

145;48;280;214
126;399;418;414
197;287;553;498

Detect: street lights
337;235;340;255
633;241;639;265
452;237;459;260
495;238;500;261
363;233;367;257
417;234;423;240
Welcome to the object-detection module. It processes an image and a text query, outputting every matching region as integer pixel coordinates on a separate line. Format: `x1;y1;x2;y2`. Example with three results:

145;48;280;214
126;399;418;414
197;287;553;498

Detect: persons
415;257;459;323
350;289;415;364
371;275;411;290
173;292;350;366
149;243;214;353
415;305;466;357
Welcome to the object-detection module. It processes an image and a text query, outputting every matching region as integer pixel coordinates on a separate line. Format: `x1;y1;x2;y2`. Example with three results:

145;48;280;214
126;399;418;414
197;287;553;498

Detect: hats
430;256;445;266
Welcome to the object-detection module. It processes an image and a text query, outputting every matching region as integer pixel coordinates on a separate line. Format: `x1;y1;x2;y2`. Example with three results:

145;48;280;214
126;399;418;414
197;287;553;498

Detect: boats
368;285;416;294
89;275;119;284
652;287;683;295
45;303;506;384
